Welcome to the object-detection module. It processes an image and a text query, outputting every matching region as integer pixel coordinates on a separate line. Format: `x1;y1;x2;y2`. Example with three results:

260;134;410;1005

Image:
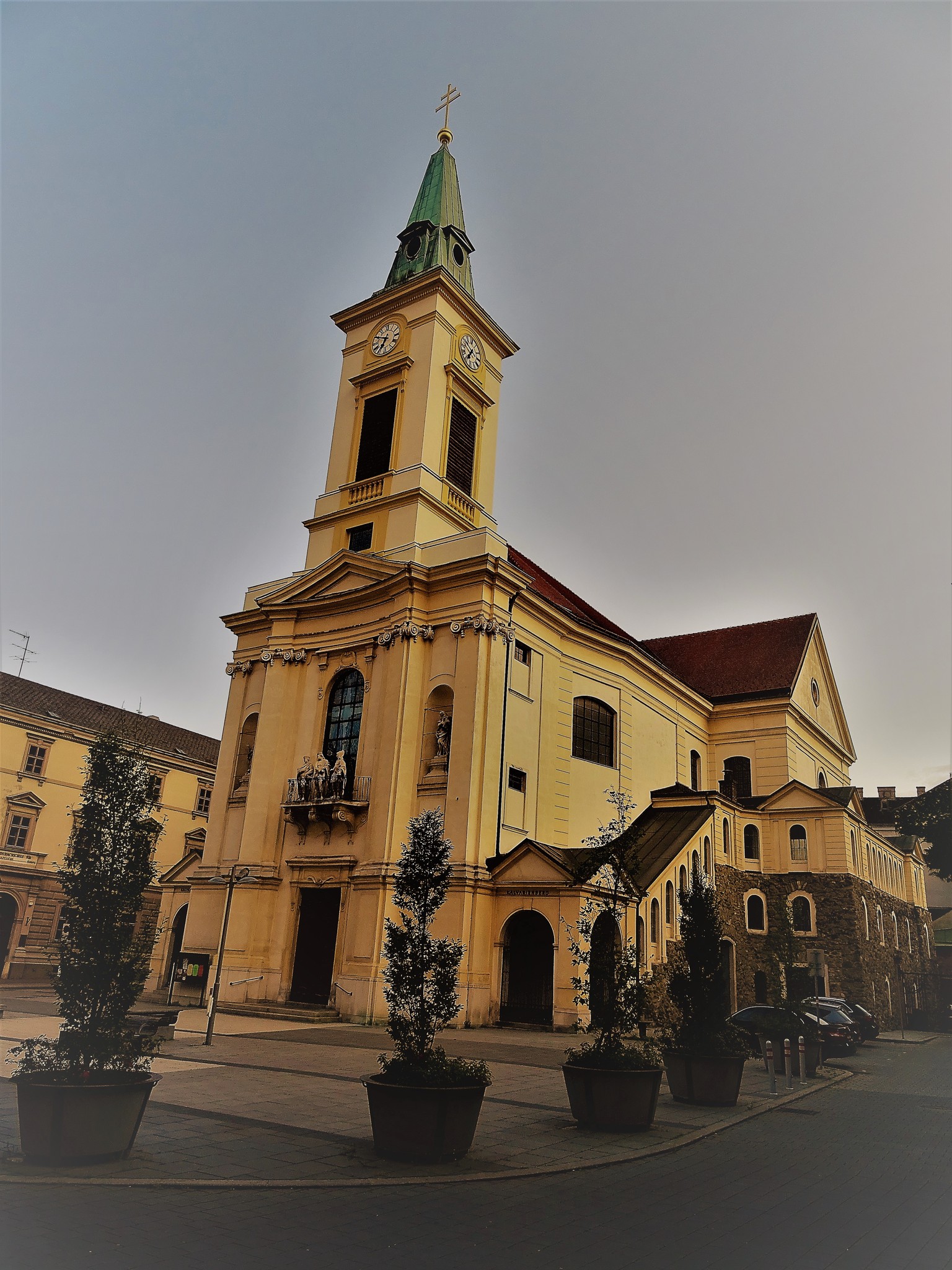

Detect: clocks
457;329;483;373
370;320;403;357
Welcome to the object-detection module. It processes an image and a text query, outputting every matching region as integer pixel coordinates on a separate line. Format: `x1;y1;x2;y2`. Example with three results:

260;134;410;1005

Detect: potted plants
660;865;750;1110
566;787;665;1138
359;808;497;1165
3;719;174;1162
756;893;825;1077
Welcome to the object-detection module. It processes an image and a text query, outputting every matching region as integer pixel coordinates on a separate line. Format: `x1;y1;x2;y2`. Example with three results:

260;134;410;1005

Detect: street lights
205;866;261;1046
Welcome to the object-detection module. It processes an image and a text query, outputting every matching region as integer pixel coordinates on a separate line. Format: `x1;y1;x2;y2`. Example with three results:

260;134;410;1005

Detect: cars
796;996;880;1051
725;1005;853;1065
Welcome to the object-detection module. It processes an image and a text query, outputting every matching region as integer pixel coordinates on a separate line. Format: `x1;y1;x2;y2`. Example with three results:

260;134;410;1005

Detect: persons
295;750;347;801
435;710;452;756
246;742;254;775
